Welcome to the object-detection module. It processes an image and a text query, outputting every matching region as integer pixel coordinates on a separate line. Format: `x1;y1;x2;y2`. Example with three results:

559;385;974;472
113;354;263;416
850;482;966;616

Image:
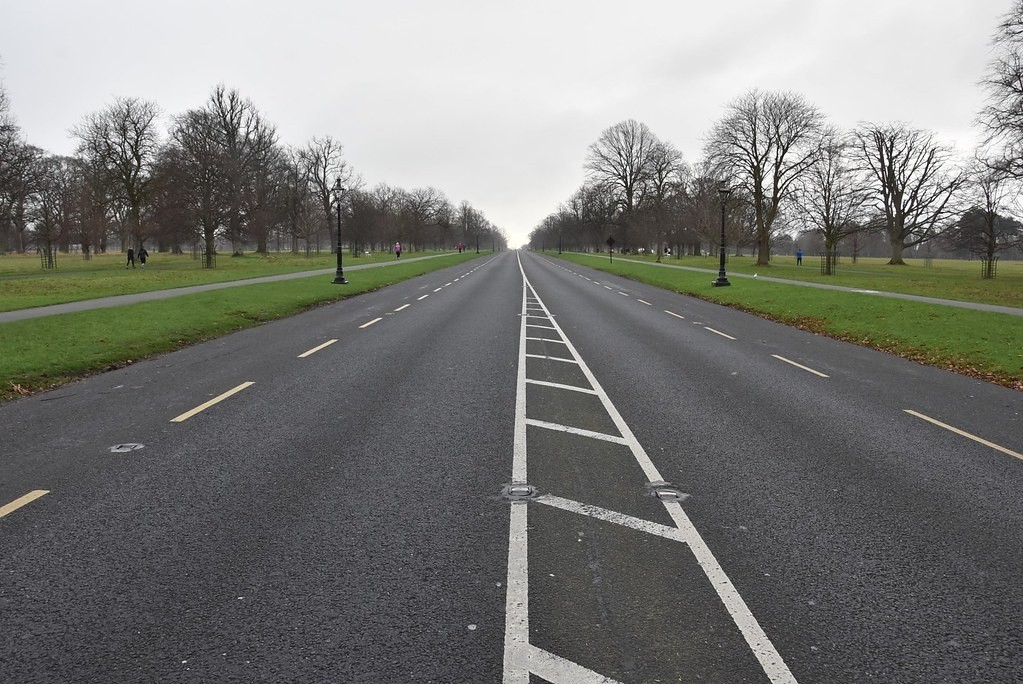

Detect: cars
700;250;710;256
637;248;655;254
621;249;630;254
608;248;616;253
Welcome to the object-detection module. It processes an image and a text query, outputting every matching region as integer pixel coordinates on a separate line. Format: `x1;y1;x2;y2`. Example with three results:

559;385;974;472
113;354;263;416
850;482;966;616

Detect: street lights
331;178;348;284
714;180;730;286
558;236;562;254
476;235;479;254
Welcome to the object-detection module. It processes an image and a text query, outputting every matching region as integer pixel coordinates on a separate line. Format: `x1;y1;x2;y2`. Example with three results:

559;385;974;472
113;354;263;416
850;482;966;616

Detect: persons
125;246;136;270
458;243;463;253
797;249;803;266
395;241;401;260
138;246;149;266
